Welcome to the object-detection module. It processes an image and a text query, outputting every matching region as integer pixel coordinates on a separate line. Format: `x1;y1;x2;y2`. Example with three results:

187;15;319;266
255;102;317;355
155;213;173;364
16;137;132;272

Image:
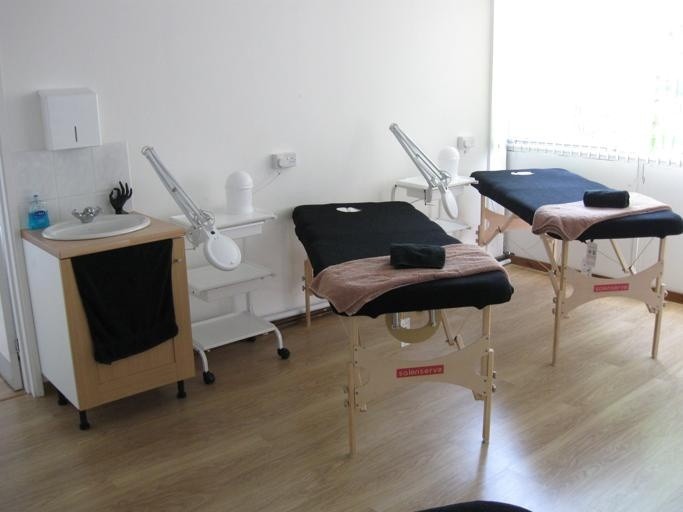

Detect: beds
292;201;515;452
470;169;683;367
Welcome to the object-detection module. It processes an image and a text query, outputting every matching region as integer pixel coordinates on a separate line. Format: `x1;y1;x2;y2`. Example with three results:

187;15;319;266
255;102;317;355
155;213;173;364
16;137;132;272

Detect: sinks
43;213;151;240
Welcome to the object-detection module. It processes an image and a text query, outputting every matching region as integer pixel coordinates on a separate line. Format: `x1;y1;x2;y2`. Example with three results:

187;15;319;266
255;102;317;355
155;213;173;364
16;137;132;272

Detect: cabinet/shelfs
19;209;290;430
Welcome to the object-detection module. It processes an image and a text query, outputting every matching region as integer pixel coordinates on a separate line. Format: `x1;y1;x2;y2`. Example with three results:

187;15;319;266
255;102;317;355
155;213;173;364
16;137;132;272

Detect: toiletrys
27;193;51;229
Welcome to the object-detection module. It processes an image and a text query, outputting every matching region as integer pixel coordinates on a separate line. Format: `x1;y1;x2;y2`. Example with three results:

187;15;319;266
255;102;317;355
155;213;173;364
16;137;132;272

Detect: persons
110;181;133;216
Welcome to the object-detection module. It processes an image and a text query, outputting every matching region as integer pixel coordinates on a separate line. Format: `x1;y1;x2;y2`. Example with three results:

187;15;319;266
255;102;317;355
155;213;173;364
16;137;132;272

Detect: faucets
70;206;100;222
